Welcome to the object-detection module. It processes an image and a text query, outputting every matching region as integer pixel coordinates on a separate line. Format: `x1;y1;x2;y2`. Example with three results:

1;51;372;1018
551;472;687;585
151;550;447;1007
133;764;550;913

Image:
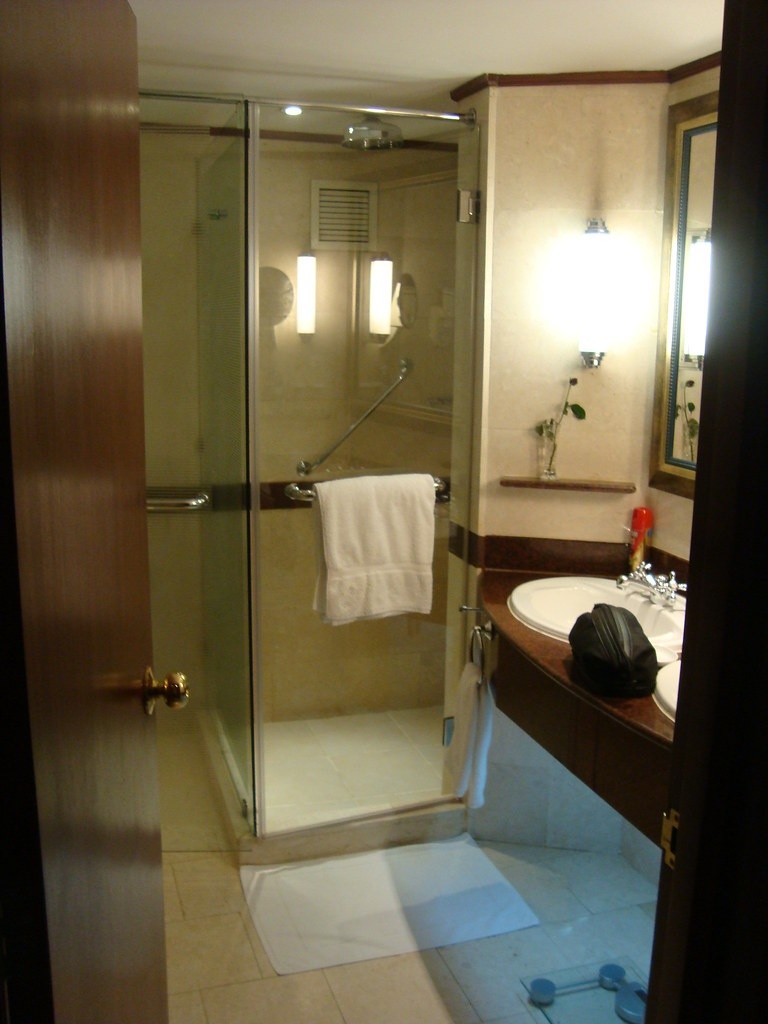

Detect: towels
237;829;541;976
314;473;436;628
440;660;495;810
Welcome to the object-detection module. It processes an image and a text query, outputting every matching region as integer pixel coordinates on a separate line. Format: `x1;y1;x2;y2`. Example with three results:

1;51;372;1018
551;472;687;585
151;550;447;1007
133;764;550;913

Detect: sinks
508;573;686;651
651;659;682;725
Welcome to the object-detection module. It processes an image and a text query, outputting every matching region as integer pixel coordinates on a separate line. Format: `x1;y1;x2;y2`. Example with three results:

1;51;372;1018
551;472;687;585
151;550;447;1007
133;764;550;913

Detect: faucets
615;574;667;600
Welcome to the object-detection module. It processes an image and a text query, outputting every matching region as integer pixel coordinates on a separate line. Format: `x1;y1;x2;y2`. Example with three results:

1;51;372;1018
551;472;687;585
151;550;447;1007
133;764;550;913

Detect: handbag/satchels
569;603;659;698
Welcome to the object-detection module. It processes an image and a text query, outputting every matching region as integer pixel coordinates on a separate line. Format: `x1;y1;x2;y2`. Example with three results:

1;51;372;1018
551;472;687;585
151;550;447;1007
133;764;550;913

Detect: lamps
369;251;392;344
295;248;317;336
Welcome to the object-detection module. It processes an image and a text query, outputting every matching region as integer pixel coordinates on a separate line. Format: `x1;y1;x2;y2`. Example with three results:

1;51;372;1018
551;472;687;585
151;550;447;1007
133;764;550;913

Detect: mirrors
648;90;736;502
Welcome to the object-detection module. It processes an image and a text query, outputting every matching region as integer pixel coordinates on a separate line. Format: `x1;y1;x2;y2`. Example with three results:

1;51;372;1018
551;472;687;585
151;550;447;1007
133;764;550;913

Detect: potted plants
535;377;586;481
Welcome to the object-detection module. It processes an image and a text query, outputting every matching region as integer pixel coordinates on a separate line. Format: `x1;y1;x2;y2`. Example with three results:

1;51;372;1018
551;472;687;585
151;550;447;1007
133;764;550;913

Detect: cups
536;437;558;481
